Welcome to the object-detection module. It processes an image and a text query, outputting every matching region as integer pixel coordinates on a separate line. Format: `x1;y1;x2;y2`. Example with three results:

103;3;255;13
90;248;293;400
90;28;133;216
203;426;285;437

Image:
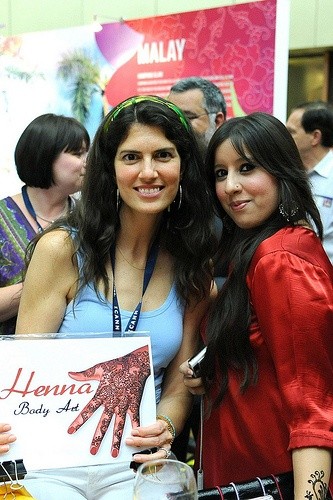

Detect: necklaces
35;206;66;224
116;246;146;270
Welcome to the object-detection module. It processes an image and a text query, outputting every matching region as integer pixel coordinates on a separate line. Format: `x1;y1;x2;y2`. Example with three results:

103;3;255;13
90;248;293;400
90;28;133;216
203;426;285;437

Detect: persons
167;77;228;291
0;94;219;500
177;112;333;500
0;113;90;335
67;345;151;458
285;101;333;265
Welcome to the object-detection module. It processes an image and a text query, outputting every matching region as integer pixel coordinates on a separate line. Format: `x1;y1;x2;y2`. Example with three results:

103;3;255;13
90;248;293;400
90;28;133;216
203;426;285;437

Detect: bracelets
164;416;176;439
156;415;174;445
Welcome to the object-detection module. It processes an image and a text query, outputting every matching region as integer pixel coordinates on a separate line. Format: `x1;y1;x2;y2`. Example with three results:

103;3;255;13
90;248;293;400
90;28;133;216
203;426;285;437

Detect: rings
158;448;171;459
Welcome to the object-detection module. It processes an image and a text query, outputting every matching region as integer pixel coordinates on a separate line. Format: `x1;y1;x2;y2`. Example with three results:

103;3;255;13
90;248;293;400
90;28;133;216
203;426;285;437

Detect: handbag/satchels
167;470;332;500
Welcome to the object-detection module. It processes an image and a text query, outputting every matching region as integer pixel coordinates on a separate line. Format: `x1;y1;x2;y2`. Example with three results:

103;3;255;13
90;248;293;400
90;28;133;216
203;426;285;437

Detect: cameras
188;346;207;387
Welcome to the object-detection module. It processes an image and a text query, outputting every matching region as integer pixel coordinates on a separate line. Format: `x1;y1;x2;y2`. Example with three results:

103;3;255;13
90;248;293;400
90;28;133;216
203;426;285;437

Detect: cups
132;459;198;500
